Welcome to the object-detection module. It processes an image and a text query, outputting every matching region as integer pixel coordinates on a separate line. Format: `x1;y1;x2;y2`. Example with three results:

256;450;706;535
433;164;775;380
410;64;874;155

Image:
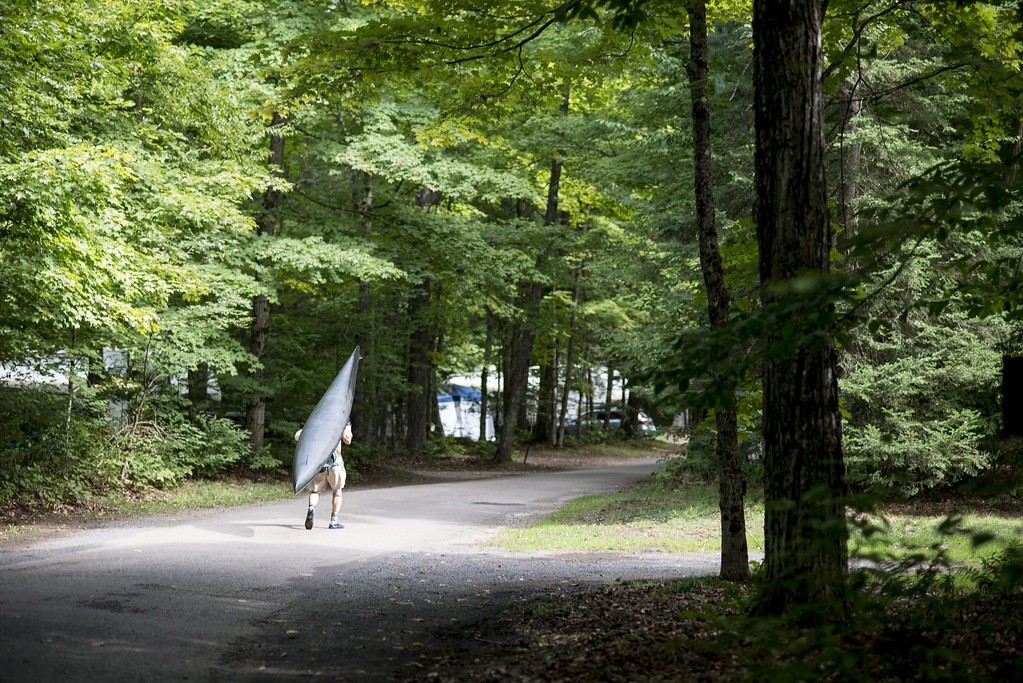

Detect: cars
559;410;656;441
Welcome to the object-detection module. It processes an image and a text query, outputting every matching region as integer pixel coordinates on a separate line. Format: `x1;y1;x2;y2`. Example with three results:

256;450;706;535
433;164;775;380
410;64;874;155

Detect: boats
291;343;361;498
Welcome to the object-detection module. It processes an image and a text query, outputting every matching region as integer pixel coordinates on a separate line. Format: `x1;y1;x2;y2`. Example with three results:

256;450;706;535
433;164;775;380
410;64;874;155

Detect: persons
295;414;353;530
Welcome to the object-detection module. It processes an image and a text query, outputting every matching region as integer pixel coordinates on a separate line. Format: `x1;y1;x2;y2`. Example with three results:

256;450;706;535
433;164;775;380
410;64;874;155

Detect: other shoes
329;524;343;529
305;507;314;530
